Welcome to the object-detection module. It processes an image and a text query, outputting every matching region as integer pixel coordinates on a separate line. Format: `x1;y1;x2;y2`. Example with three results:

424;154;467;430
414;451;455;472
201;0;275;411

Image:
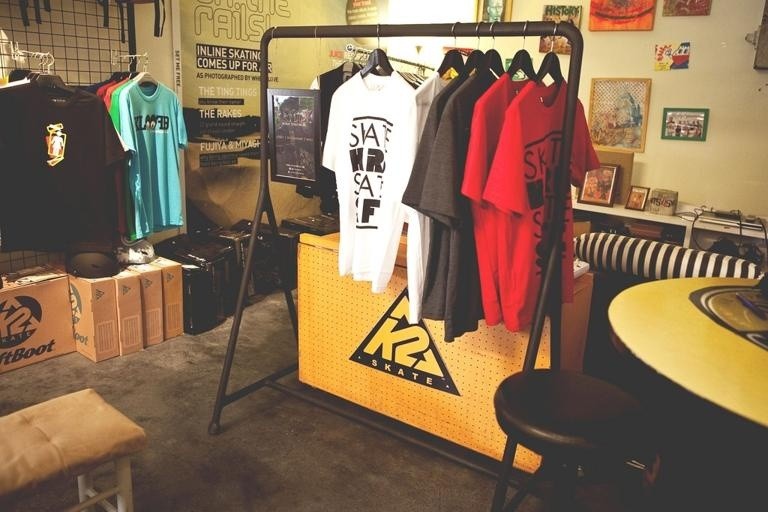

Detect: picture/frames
263;87;322;187
576;76;709;213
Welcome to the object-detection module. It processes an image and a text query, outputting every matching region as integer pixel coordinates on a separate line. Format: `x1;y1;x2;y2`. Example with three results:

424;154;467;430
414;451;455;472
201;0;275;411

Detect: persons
666;42;690;69
586;169;597;196
600;169;612;198
485;0;503;21
666;114;702;137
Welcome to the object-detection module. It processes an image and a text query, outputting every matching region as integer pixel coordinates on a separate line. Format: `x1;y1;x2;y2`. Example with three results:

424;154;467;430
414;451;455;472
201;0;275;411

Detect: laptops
697;211;763;231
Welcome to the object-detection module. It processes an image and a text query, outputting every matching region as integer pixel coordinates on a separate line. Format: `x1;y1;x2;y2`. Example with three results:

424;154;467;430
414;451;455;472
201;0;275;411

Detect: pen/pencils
735;292;766;320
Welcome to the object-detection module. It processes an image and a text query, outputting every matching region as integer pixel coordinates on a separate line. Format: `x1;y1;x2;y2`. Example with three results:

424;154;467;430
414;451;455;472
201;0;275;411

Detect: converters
715;209;737;219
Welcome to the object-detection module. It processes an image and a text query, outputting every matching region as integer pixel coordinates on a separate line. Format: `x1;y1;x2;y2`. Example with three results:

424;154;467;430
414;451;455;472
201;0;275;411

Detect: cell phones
746;214;757;221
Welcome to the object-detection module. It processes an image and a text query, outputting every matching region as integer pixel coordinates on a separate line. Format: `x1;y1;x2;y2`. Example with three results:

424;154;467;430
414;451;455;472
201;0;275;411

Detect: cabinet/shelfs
0;47;179;119
317;19;583;113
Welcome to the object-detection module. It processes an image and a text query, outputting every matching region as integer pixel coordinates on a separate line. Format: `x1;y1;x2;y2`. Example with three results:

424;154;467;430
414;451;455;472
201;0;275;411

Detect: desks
607;276;767;511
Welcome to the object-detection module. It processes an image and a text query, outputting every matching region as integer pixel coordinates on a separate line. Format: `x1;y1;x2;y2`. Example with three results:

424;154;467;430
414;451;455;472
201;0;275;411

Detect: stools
1;387;146;512
489;368;641;512
